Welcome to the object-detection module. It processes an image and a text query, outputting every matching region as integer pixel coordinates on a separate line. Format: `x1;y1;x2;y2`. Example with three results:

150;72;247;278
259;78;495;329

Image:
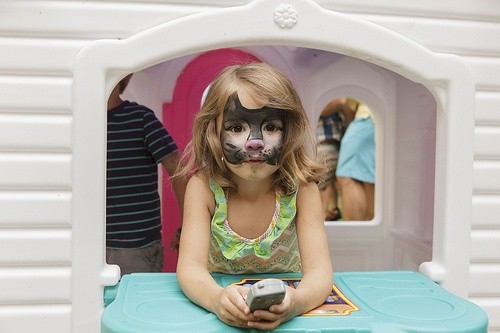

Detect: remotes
246;278;286;313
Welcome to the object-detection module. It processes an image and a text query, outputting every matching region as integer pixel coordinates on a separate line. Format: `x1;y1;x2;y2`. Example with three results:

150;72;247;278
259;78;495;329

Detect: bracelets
175;227;182;237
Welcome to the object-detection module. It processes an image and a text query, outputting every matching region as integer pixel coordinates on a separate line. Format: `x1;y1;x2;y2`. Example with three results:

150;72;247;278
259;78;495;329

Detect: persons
106;73;191;281
176;62;334;330
314;96;380;222
